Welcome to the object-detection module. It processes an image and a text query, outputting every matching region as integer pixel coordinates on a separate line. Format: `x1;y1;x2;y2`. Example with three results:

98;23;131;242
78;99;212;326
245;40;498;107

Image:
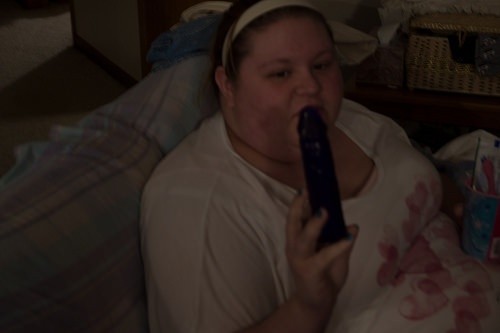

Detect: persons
139;1;412;333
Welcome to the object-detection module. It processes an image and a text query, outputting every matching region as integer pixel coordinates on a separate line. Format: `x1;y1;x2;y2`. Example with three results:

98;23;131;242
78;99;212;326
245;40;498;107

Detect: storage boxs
405;12;499;98
331;35;404;89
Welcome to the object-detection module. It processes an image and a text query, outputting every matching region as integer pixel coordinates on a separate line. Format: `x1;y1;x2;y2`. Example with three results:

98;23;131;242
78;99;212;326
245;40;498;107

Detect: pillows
1;57;226;331
